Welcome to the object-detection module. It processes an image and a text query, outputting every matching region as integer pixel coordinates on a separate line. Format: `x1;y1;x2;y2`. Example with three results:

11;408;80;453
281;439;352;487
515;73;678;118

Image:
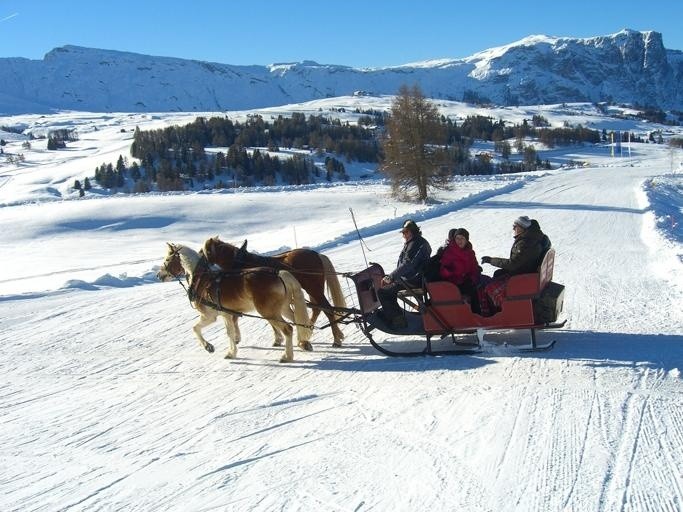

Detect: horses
198;234;347;348
156;241;313;364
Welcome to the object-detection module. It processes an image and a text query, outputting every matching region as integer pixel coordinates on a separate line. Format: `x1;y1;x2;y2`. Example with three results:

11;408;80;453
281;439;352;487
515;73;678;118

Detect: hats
514;216;532;229
530;219;540;230
399;220;418;234
449;229;457;239
454;228;469;240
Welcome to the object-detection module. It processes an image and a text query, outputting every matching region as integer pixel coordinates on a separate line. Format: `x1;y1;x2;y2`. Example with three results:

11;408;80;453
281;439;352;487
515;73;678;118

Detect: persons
477;216;541;315
375;219;431;329
440;228;483;315
531;219;551;267
430;228;456;267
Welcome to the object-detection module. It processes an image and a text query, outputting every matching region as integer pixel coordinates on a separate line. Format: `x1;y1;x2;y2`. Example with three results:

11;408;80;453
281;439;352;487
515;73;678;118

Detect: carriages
154;232;568;360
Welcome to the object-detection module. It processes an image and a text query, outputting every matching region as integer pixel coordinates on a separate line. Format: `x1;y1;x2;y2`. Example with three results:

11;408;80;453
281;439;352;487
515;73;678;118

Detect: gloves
481;256;491;264
382;275;391;285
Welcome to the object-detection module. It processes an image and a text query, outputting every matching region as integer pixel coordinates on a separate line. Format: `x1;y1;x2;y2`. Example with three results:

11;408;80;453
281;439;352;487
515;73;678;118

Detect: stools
396;288;427;318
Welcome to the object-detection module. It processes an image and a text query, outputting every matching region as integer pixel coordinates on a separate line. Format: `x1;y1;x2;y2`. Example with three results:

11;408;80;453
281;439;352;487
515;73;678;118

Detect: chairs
422;247;555;334
